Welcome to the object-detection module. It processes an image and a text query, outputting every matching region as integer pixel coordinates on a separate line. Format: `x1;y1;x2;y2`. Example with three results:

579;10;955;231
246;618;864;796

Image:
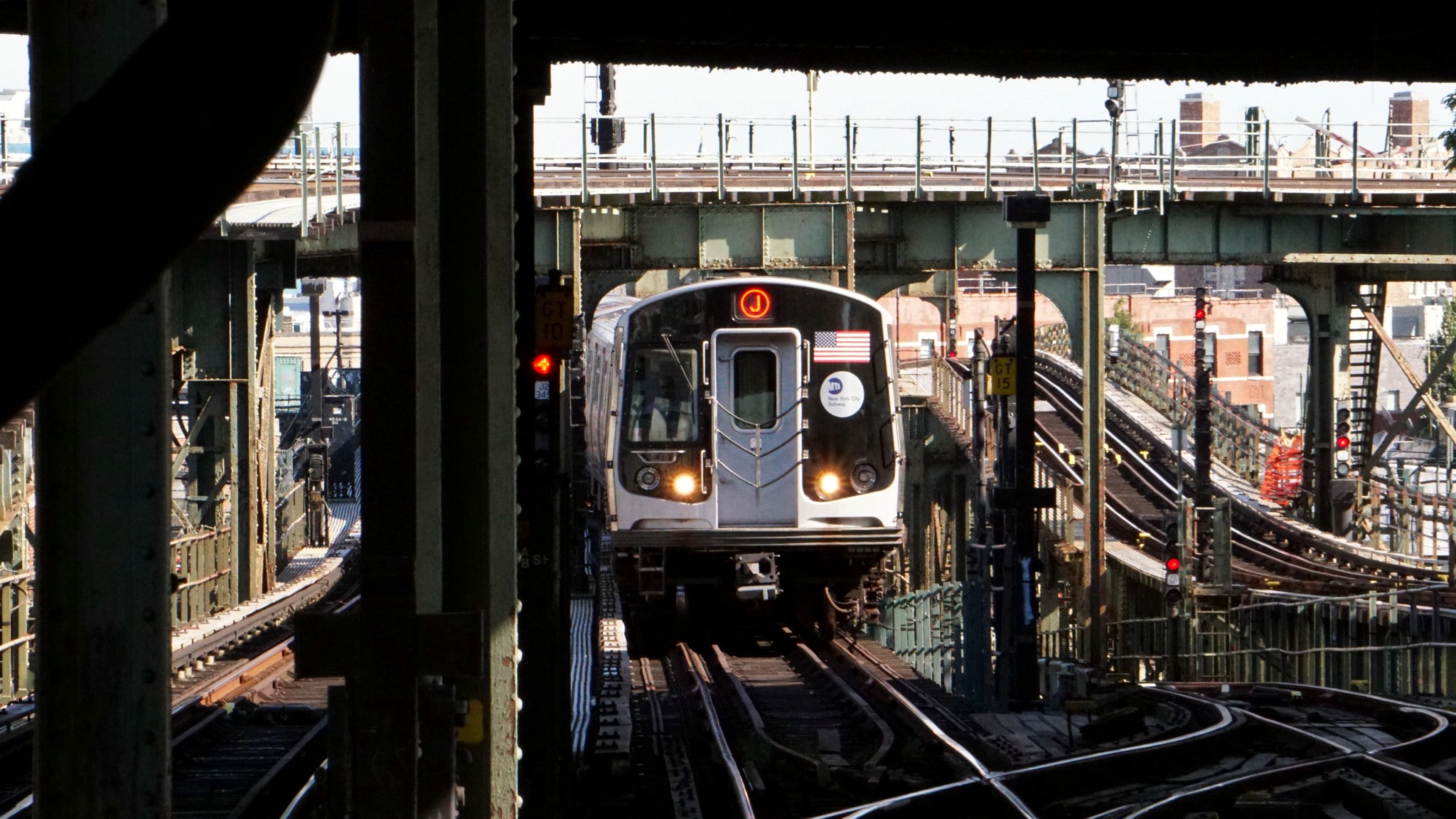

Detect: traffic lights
1105;77;1123;117
1194;286;1206;369
1163;514;1183;618
1337;408;1350;477
531;348;558;402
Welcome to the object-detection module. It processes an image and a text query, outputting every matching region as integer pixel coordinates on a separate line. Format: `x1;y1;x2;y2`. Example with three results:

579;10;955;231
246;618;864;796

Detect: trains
579;264;908;640
